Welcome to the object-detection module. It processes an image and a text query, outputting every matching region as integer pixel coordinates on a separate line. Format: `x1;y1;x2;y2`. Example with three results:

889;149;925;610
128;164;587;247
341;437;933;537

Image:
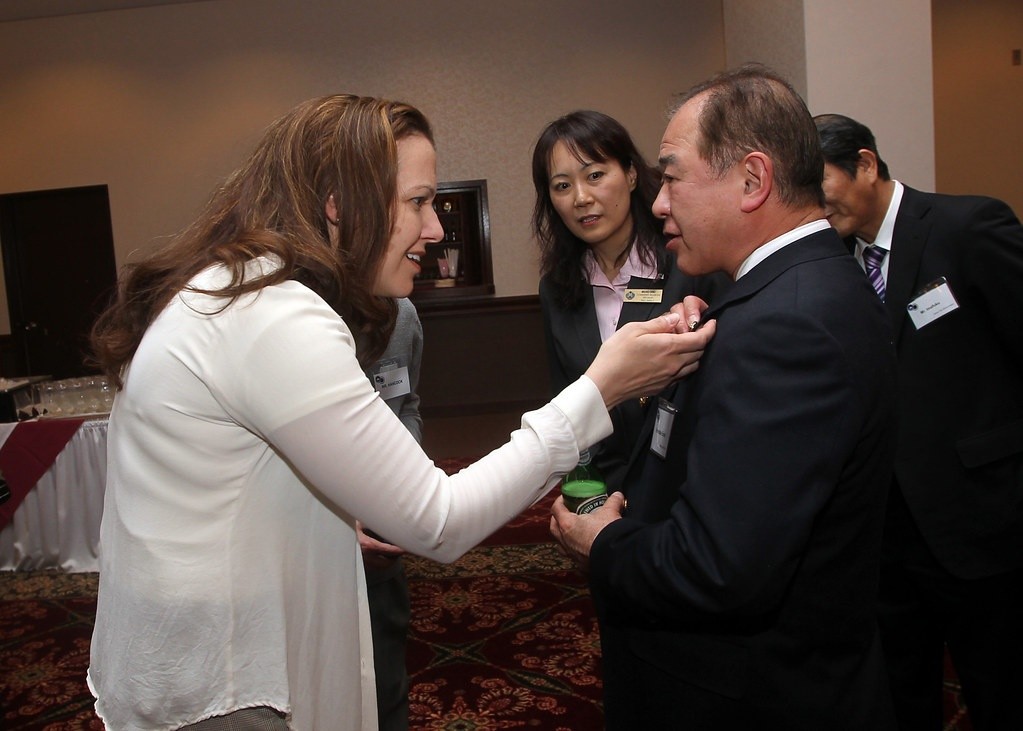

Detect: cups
12;375;114;421
437;258;449;278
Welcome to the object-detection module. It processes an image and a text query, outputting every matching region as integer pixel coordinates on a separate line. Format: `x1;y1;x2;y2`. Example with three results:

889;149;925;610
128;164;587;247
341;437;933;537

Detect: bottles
559;447;609;515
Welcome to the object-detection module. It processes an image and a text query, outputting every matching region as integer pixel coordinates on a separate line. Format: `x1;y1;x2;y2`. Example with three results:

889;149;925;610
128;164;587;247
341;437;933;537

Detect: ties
861;245;888;304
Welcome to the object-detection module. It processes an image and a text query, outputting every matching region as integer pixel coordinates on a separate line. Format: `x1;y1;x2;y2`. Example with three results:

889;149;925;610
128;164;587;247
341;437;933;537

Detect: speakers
1;184;118;399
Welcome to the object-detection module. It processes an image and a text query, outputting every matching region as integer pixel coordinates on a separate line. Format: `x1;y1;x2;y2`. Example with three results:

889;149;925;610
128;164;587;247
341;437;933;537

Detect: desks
0;411;110;576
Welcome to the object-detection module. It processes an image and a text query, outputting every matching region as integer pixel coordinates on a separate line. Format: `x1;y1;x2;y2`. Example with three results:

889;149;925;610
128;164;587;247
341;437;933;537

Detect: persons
550;67;898;730
355;295;426;731
812;114;1023;731
82;87;717;731
530;110;733;492
